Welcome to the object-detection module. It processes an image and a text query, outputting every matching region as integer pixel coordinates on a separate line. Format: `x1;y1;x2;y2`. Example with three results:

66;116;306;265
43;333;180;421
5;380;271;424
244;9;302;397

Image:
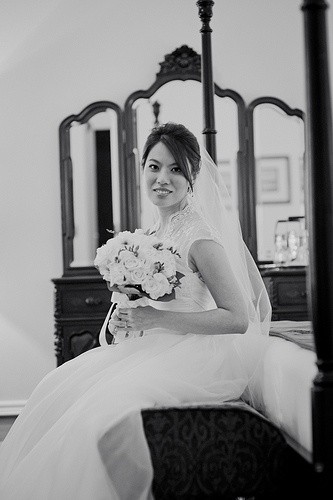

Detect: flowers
92;228;182;343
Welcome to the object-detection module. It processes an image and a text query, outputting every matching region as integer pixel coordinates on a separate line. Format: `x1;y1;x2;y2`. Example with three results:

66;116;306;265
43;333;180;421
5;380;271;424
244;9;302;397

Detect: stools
140;403;324;500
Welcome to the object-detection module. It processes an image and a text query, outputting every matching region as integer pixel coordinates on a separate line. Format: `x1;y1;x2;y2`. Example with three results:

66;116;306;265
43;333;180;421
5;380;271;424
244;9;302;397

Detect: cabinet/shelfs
51;273;310;368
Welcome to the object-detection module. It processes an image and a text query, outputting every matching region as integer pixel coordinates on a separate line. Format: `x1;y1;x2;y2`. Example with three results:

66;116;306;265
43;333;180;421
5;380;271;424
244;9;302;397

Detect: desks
239;321;316;461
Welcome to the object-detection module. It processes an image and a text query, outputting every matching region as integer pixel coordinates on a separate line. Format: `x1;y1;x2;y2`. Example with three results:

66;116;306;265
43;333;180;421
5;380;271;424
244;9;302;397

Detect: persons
0;123;249;500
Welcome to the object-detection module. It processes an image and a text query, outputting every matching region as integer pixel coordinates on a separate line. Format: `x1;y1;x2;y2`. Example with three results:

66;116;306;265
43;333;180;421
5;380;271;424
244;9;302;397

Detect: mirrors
59;45;309;278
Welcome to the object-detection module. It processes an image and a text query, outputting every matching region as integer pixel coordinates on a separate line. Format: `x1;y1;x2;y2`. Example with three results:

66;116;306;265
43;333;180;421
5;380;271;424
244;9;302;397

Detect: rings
125;322;127;329
